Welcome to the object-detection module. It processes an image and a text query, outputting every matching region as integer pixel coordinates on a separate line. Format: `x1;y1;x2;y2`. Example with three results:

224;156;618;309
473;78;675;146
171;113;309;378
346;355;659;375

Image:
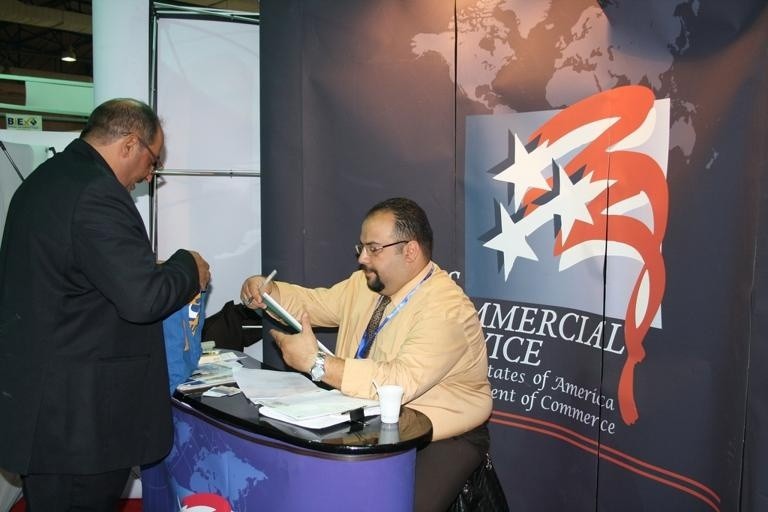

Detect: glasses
354;240;410;257
118;130;164;173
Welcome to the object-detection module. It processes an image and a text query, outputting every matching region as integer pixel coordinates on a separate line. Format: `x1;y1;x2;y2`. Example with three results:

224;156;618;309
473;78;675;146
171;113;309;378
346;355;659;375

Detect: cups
377;424;400;445
378;385;405;423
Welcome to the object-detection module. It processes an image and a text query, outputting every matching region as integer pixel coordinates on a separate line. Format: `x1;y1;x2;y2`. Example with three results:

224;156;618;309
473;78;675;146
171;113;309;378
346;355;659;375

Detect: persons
1;97;213;512
238;198;495;512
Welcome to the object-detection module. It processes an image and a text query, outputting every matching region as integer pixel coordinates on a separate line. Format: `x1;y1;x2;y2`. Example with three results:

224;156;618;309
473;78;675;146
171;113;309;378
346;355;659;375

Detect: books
261;292;336;357
176;339;382;431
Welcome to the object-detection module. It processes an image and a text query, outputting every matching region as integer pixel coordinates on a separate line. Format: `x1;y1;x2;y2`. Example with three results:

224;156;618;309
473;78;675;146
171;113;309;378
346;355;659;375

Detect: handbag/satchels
162;286;208;397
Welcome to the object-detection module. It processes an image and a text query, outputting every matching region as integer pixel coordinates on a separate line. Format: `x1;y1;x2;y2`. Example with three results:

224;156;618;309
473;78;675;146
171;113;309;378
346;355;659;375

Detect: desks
141;347;433;511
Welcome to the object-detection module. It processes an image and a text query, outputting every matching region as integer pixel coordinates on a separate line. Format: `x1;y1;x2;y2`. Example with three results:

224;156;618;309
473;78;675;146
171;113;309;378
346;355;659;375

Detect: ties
354;296;391;359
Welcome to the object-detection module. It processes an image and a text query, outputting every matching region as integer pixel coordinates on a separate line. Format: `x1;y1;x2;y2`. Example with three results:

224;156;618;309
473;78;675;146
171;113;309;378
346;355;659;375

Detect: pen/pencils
247;270;277;305
